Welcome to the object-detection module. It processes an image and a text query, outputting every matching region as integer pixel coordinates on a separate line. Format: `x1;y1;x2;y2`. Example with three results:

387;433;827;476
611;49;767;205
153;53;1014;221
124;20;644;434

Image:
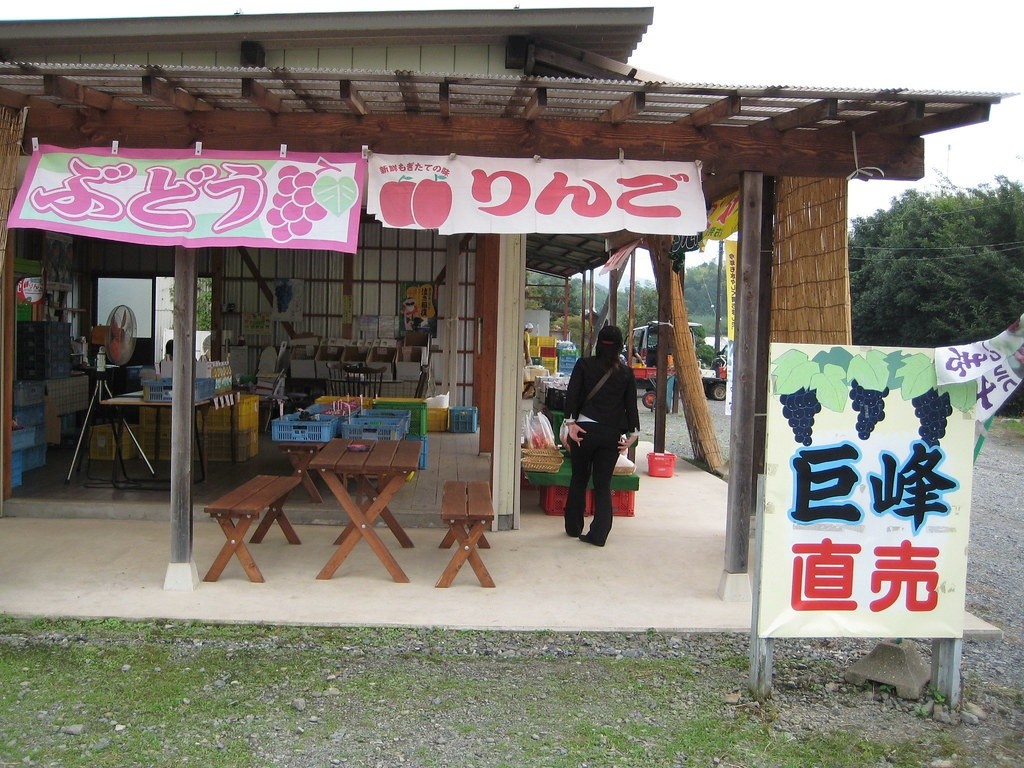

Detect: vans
624;320;703;368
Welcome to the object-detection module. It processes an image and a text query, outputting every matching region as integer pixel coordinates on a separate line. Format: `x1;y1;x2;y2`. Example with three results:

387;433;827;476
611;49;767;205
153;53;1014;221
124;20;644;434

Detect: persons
524;322;533;366
563;326;640;547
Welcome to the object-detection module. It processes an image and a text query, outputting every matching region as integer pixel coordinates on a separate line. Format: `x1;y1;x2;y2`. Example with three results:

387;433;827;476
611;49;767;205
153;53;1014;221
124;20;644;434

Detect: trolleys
641;368;675;414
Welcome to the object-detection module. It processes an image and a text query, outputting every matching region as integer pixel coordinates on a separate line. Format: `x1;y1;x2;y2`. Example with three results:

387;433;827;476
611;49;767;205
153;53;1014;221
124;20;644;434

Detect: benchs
434;480;497;588
202;475;300;584
279;441;336;503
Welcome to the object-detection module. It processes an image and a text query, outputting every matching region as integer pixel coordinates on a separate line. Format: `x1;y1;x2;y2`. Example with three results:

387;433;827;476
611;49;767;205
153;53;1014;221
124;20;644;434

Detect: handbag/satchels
559;418;571;456
524;410;556;450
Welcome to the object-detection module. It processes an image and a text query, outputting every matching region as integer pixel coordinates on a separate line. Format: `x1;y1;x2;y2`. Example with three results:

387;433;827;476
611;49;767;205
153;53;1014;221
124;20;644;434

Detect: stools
263;395;288;433
286;392;307;414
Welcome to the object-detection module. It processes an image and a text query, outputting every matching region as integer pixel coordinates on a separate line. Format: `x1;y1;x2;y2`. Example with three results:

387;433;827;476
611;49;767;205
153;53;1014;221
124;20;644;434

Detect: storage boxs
16;321;72;379
143;377;216;403
451;406;478;433
125;366;155;381
646;453;677;478
88;423;139;461
139;394;259;464
270;395;428;471
426;407;449;432
11;378;48;487
289;331;435;382
632;367;658;379
522;336;639;516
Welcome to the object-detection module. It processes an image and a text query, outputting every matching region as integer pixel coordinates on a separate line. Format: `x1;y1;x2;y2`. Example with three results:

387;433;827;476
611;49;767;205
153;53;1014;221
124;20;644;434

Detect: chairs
248;369;288;433
326;361;364;396
414;365;430;399
343;364;387;398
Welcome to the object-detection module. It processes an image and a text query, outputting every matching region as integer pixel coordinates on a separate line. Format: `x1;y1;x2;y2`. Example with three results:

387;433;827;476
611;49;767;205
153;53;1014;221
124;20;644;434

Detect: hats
524;323;533;330
598;326;623;345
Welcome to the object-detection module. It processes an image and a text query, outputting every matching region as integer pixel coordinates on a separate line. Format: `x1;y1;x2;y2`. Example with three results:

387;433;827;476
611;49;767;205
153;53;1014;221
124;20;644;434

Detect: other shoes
579;535;604;546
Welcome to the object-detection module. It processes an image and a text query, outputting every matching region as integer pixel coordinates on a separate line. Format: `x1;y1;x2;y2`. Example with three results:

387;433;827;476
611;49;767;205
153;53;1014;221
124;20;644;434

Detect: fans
64;305;156;488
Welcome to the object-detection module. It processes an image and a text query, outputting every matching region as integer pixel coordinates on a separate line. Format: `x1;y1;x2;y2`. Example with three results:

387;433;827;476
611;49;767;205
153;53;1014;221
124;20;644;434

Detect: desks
309;437;422;583
76;365;120;427
328;378;403;397
42;375;88;449
101;387;242;490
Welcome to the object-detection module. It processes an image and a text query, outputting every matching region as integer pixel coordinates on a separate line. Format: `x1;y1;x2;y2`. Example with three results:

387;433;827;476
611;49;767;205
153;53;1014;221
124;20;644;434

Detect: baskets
612;454;636;476
521;448;564;473
143;377;215;403
271;403;411;442
